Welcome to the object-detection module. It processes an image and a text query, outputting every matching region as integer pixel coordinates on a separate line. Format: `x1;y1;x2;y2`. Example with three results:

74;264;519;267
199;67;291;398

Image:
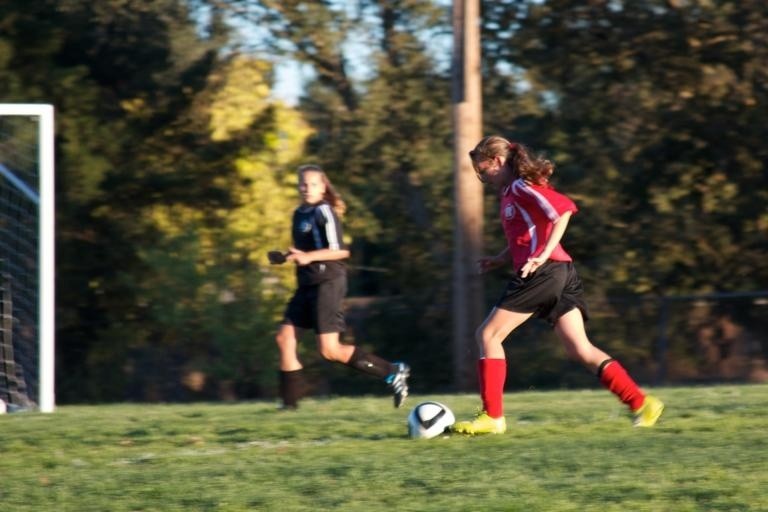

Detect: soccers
408;402;454;438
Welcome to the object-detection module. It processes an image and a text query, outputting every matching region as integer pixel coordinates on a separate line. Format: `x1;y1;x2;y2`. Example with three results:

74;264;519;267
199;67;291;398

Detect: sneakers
630;395;664;428
449;410;507;436
385;362;410;408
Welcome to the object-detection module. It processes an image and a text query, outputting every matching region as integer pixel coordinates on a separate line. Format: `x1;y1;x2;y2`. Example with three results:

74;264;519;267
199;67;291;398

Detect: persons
274;164;413;409
444;135;666;437
